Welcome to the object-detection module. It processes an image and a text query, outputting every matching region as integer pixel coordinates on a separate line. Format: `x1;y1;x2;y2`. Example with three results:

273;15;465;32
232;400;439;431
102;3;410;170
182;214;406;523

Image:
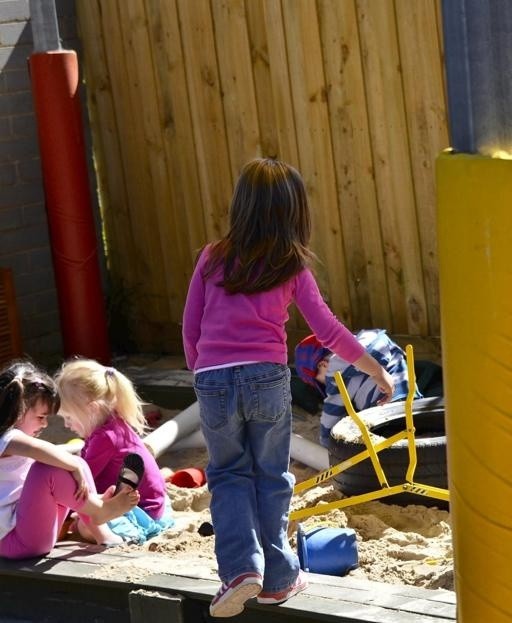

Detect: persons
0;363;141;561
182;158;396;617
294;330;424;446
54;359;169;543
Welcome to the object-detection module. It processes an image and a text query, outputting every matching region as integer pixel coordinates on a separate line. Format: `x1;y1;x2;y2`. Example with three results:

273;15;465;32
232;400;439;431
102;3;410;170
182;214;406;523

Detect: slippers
111;454;145;503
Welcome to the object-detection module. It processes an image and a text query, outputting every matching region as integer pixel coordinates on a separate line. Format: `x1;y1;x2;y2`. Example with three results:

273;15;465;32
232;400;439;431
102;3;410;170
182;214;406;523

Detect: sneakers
209;571;263;617
256;569;308;604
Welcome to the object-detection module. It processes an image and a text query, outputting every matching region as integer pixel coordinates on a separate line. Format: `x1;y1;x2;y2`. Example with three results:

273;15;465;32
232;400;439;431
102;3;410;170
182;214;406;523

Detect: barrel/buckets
297;524;359;576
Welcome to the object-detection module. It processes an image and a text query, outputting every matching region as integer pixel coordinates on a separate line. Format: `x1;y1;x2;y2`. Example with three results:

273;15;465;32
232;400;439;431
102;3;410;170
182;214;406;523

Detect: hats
293;333;331;399
